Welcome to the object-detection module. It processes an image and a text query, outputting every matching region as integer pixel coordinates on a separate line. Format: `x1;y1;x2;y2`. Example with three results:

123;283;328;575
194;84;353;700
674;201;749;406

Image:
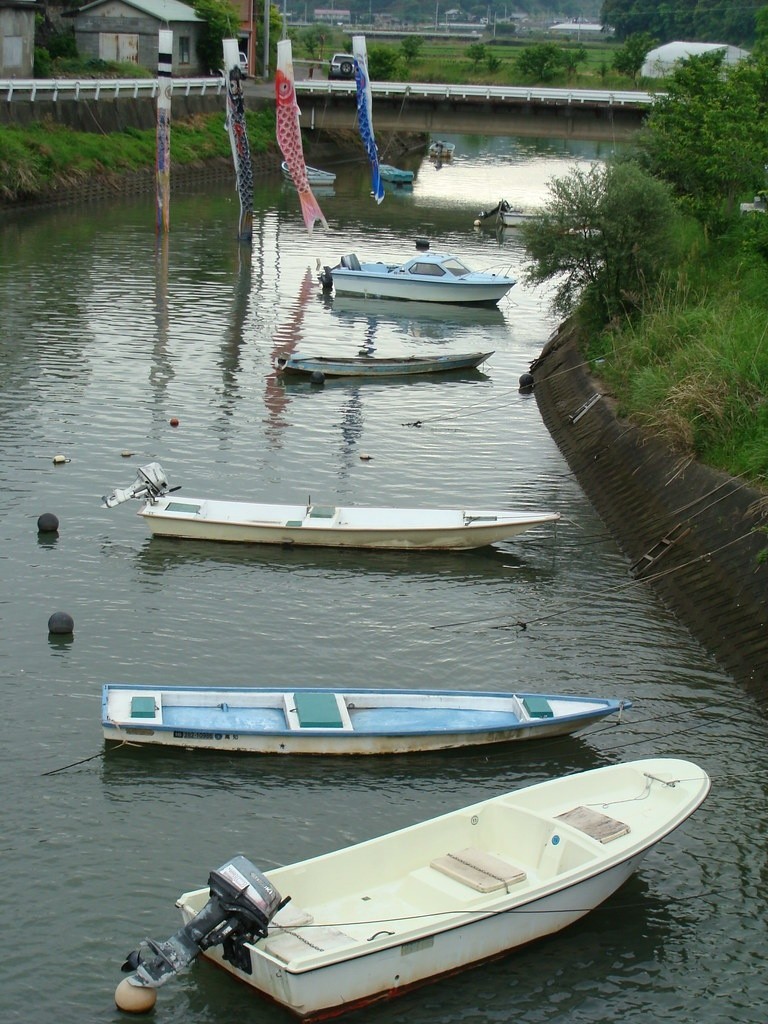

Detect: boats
281;157;337;185
316;252;518;303
481;199;606;237
273;350;499;376
101;685;637;756
379;163;416;184
99;461;563;552
428;141;455;159
115;755;711;1017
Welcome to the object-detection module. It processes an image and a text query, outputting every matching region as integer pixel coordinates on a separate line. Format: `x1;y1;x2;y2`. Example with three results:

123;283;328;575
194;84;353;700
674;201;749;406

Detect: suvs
328;53;357;79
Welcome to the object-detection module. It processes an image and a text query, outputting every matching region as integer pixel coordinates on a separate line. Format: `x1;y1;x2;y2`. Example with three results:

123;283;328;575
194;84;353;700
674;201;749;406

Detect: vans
237;51;249;79
